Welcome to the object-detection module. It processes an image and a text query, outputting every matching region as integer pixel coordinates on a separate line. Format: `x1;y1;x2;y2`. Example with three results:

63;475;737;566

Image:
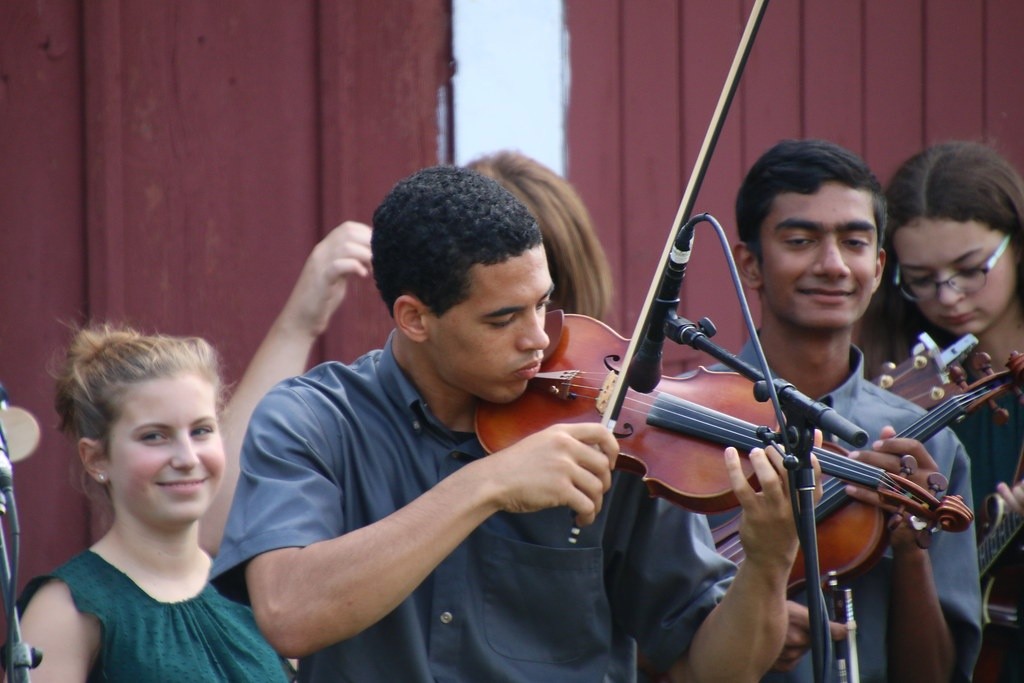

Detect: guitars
866;329;983;426
975;490;1023;630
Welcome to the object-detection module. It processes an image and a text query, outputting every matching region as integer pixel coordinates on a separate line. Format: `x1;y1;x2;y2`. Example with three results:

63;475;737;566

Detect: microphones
630;223;695;394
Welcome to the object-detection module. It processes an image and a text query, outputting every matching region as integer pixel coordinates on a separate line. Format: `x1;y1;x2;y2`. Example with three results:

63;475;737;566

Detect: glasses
895;234;1011;302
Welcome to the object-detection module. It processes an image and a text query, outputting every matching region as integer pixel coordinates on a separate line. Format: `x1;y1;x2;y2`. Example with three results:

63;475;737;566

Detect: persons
20;138;1023;683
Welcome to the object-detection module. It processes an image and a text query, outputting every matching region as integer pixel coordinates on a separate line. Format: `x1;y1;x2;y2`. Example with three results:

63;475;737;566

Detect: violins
709;348;1023;598
471;308;983;550
